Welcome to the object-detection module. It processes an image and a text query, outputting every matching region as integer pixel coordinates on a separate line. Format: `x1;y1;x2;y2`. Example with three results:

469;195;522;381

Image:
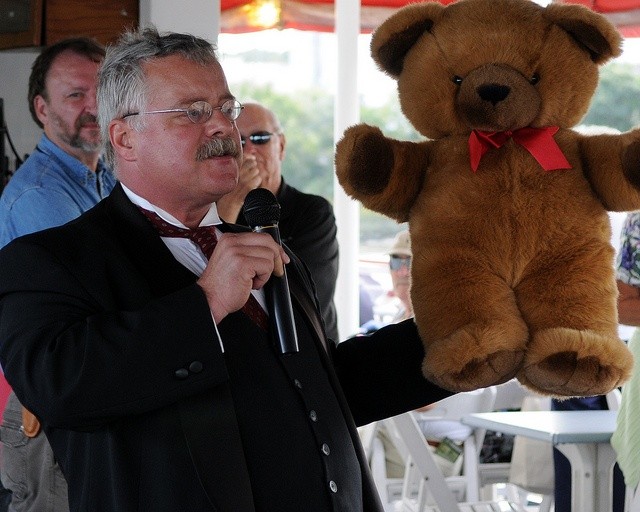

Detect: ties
139;206;274;336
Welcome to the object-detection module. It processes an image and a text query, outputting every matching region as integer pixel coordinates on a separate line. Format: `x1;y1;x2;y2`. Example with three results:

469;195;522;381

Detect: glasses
241;131;284;146
390;254;411;270
119;100;245;125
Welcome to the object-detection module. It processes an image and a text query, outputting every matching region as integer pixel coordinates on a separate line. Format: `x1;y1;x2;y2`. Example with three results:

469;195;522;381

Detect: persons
215;100;340;349
346;231;417;340
2;35;121;511
3;27;459;511
616;212;634;327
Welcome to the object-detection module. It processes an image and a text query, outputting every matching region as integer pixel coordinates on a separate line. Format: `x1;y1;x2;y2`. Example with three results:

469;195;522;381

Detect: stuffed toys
333;0;640;398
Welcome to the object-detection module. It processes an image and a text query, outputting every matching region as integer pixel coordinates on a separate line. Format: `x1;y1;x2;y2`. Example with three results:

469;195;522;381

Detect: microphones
242;188;300;354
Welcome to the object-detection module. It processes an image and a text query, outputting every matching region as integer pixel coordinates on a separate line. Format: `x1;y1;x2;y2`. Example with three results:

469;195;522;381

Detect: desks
462;411;622;511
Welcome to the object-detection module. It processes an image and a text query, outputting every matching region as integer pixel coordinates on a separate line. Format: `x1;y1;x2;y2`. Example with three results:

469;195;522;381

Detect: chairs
392;412;530;510
